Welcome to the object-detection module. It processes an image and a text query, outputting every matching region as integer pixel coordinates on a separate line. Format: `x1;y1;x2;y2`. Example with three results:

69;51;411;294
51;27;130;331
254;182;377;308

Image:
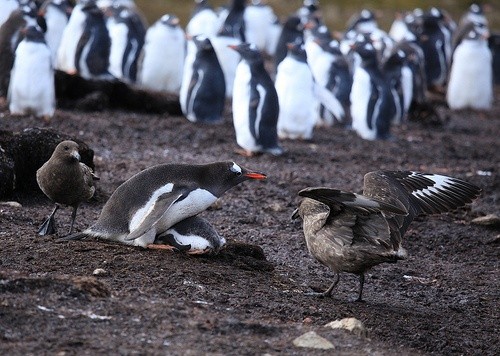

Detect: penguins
0;0;500;160
52;160;268;257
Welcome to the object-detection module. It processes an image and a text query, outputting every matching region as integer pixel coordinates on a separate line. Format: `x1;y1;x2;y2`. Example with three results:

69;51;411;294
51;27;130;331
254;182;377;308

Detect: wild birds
33;139;101;241
286;167;484;307
